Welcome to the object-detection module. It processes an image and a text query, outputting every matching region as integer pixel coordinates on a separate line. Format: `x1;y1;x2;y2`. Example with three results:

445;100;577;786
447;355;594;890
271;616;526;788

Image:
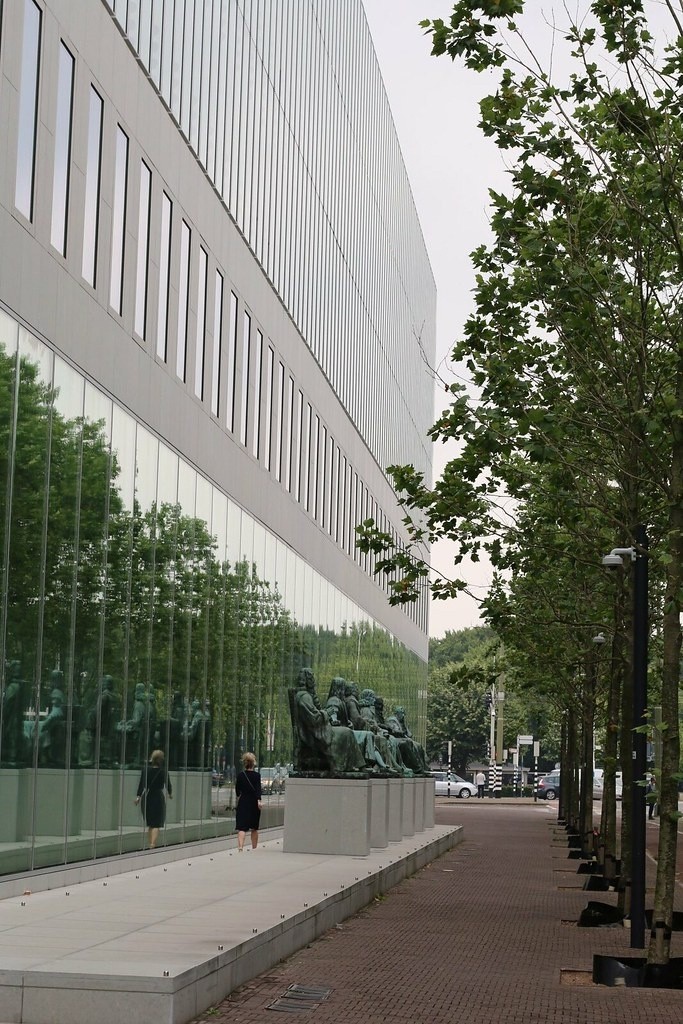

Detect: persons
389;707;431;774
235;753;261;851
295;669;413;774
4;660;212;767
477;770;485;798
648;778;657;819
135;750;173;849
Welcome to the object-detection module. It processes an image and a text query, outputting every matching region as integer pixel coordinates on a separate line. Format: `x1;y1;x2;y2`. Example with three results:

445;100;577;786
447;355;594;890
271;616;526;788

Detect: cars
537;769;654;800
430;772;478;799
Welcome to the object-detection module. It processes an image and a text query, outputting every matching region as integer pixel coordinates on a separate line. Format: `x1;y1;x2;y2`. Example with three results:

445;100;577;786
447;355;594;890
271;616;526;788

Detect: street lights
602;541;645;950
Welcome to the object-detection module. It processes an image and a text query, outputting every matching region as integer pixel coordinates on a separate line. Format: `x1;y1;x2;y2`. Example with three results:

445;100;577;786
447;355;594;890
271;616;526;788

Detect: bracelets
137;796;140;798
258;800;260;801
